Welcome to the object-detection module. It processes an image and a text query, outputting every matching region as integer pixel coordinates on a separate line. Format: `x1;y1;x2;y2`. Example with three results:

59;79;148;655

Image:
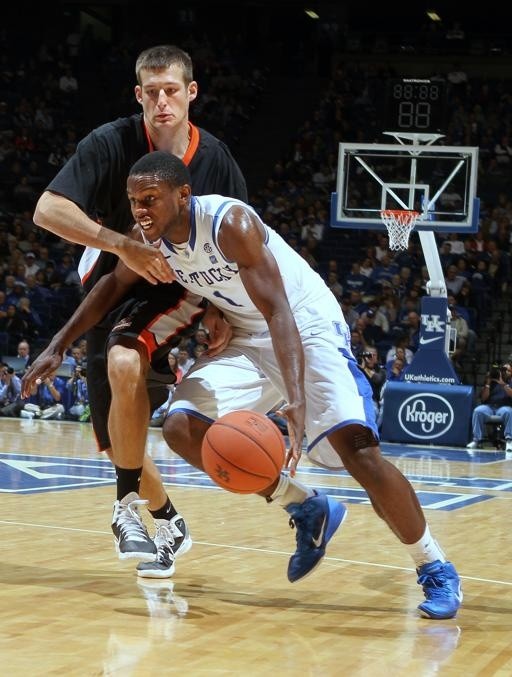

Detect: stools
482;421;506;452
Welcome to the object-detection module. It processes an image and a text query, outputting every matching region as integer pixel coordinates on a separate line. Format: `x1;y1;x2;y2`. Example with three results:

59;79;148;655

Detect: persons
19;148;462;620
1;0;511;456
34;44;251;579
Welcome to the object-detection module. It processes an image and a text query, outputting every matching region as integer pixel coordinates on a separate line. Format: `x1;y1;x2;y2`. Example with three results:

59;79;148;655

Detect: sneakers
56;412;65;421
505;438;512;452
465;440;483;449
282;486;349;586
109;488;160;566
413;557;465;623
19;409;37;419
132;510;195;580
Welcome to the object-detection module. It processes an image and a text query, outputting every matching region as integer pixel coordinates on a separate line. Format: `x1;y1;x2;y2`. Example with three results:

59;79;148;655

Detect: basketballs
201;408;286;494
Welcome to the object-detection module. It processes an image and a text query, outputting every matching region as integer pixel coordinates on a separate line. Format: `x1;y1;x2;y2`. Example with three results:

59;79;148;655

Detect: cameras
4;368;14;375
80;367;87;376
